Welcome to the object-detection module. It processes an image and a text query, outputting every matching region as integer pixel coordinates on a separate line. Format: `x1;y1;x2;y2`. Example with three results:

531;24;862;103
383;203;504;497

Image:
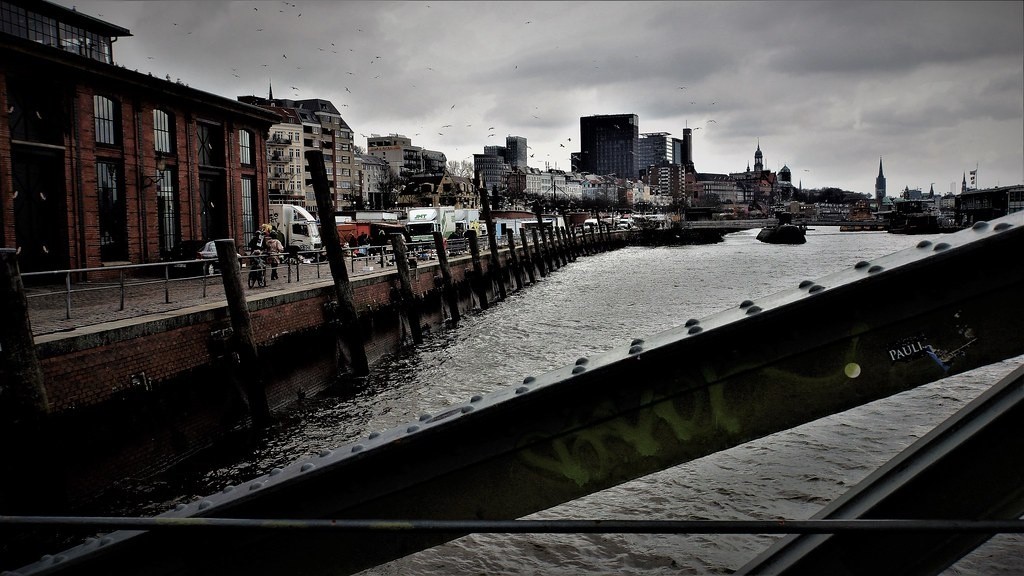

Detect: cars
162;238;242;276
583;216;632;231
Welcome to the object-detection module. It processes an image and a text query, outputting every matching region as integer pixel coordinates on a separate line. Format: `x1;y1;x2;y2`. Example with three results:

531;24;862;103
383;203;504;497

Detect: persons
345;230;389;265
247;229;285;287
447;226;477;254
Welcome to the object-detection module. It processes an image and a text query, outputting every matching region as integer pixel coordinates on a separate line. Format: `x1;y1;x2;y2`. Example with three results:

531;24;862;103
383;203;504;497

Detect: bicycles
248;260;266;289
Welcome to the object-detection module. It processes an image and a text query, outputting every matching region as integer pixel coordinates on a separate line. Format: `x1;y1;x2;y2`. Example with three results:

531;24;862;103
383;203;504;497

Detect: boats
888;199;958;235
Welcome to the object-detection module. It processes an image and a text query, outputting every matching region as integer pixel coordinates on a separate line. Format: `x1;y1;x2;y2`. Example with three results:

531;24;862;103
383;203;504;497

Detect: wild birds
172;1;381;108
72;4;77;11
676;83;719;132
553;136;638;177
114;56;190;88
414;100;539;158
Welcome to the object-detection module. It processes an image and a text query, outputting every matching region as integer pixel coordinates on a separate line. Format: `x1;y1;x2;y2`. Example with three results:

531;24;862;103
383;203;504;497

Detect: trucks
406;207;479;251
268;203;322;252
